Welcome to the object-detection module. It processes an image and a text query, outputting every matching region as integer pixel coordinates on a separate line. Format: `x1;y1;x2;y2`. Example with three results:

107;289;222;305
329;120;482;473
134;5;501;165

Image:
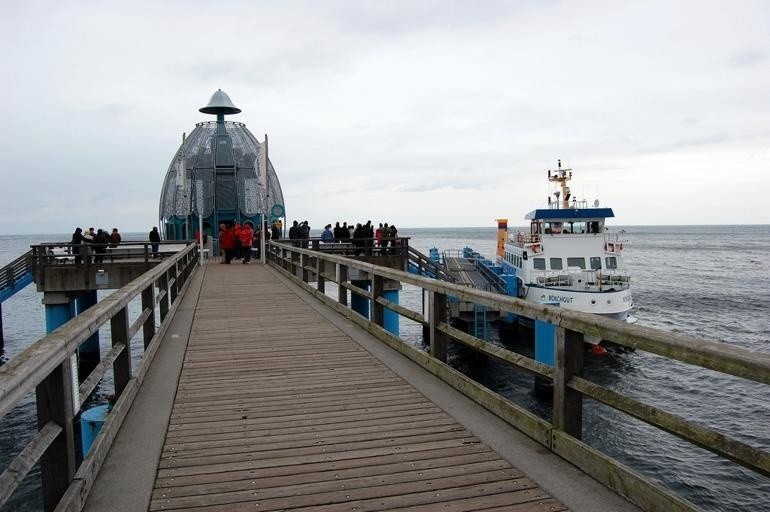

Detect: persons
196;227;203;250
219;220;403;264
149;228;160;258
71;228;121;264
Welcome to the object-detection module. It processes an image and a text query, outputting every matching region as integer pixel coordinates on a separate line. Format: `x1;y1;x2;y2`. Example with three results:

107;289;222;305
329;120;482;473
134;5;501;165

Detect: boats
496;159;639;345
